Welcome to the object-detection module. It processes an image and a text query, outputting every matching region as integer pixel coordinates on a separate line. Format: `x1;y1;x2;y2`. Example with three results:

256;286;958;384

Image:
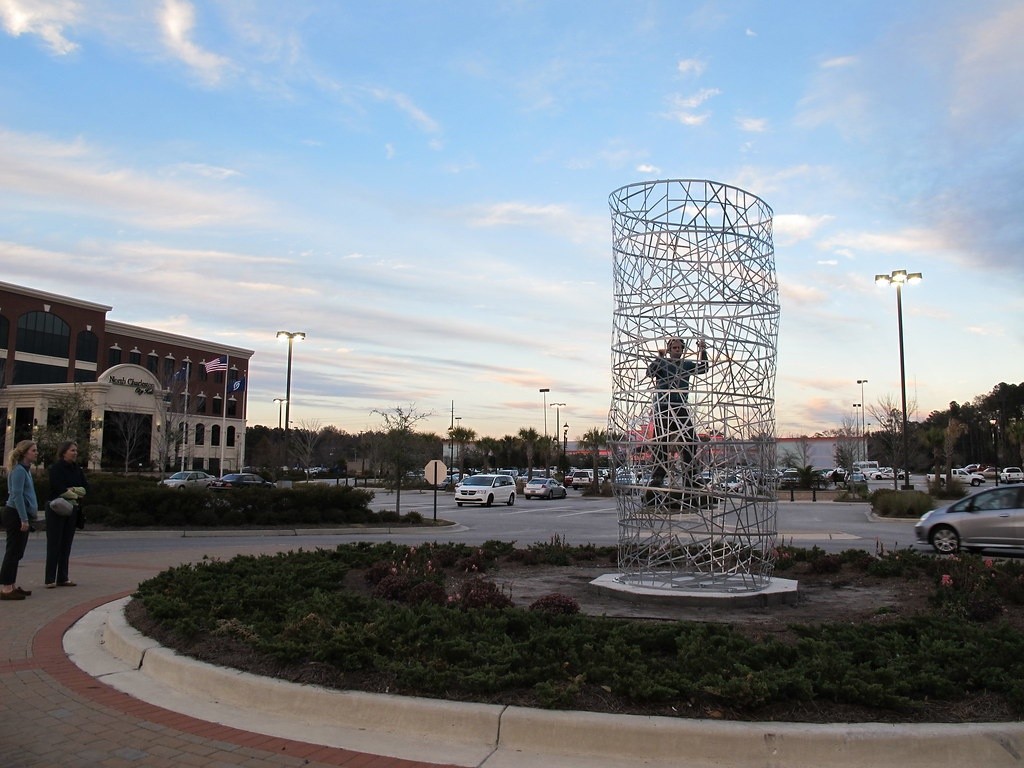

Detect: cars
209;473;276;488
158;471;216;491
455;474;516;507
914;484;1024;555
973;467;1002;479
967;465;987;474
959;465;977;473
304;461;912;500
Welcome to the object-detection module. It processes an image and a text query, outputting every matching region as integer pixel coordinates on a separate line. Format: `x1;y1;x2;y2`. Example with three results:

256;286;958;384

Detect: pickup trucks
1000;467;1024;484
927;469;986;488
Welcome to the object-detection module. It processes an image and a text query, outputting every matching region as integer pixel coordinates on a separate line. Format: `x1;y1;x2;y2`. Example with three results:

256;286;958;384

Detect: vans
853;461;879;472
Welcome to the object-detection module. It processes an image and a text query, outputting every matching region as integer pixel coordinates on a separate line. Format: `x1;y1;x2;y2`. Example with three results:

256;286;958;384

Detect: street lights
276;331;306;429
876;270;923;487
563;424;569;486
853;404;862;461
273;399;288;428
456;417;462;462
989;415;998;488
550;403;566;455
539;388;550;436
857;379;868;462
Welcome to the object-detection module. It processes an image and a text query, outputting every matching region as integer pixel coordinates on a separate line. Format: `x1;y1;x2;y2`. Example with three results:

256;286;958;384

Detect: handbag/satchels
75;504;86;528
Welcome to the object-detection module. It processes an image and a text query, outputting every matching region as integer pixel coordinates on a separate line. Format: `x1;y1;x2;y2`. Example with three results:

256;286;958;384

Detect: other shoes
683;476;701;487
57;580;76;586
45;583;58;588
16;587;31;595
652;475;665;485
0;589;25;599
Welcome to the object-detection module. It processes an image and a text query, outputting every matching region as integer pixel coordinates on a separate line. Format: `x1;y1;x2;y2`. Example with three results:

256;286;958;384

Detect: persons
0;440;38;599
831;470;839;486
44;439;86;588
985;493;1012;509
646;339;709;494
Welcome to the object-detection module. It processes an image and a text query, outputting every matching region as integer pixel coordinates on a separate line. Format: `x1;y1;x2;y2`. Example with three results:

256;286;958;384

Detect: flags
227;376;245;393
174;366;187;381
205;356;227;373
